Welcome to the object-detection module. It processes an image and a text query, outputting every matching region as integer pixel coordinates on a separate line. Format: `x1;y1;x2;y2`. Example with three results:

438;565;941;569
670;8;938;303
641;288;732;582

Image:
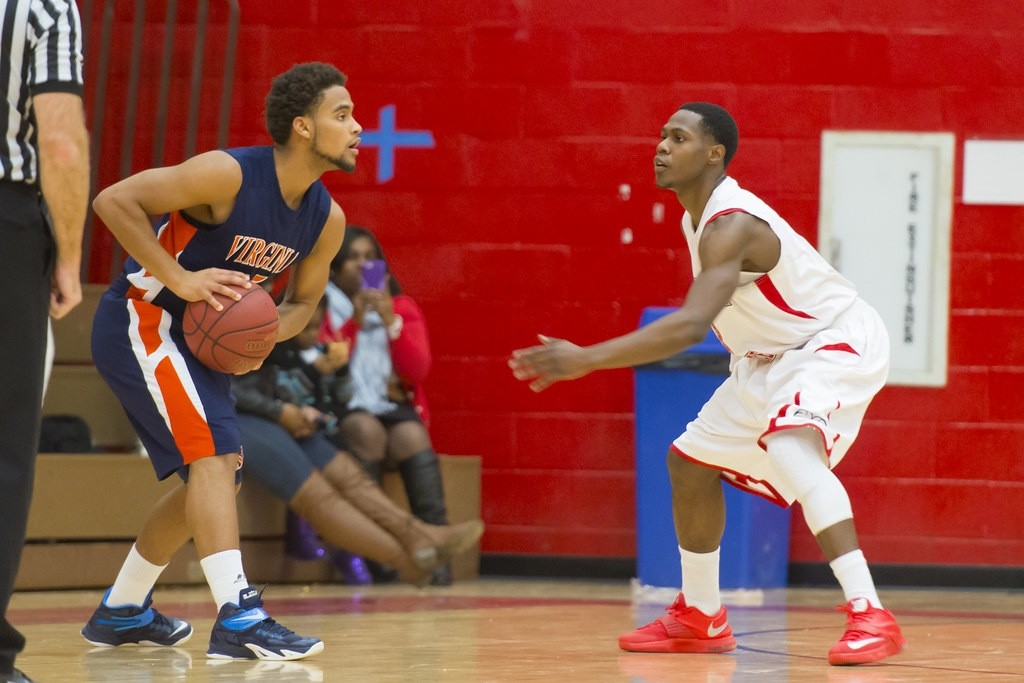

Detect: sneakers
619;590;737;654
829;597;906;664
80;583;194;650
205;581;323;662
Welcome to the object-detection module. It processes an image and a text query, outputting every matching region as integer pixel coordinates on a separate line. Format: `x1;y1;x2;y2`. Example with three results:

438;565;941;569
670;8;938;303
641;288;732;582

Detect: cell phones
361;259;386;292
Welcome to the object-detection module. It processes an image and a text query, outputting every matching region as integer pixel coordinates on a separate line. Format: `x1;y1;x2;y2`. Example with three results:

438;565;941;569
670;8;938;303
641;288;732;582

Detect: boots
350;452;399;581
288;466;452;585
324;451;484;567
400;448;453;586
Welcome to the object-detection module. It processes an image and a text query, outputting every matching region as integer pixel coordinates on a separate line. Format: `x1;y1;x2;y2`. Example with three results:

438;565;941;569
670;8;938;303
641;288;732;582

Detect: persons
511;102;906;667
88;62;362;662
0;0;89;683
232;228;483;586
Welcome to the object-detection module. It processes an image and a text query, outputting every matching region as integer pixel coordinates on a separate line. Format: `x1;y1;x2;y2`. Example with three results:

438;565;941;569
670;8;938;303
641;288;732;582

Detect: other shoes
286;508;374;582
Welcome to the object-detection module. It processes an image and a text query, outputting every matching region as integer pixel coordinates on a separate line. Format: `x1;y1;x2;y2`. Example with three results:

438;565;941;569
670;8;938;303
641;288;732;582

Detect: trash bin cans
631;306;790;592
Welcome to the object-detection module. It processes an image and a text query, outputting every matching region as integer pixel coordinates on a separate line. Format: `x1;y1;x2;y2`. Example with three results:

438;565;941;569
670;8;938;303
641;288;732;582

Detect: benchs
14;285;484;592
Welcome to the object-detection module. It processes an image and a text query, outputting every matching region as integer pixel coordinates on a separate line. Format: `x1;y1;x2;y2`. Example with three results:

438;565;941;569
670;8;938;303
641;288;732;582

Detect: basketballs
183;281;280;373
